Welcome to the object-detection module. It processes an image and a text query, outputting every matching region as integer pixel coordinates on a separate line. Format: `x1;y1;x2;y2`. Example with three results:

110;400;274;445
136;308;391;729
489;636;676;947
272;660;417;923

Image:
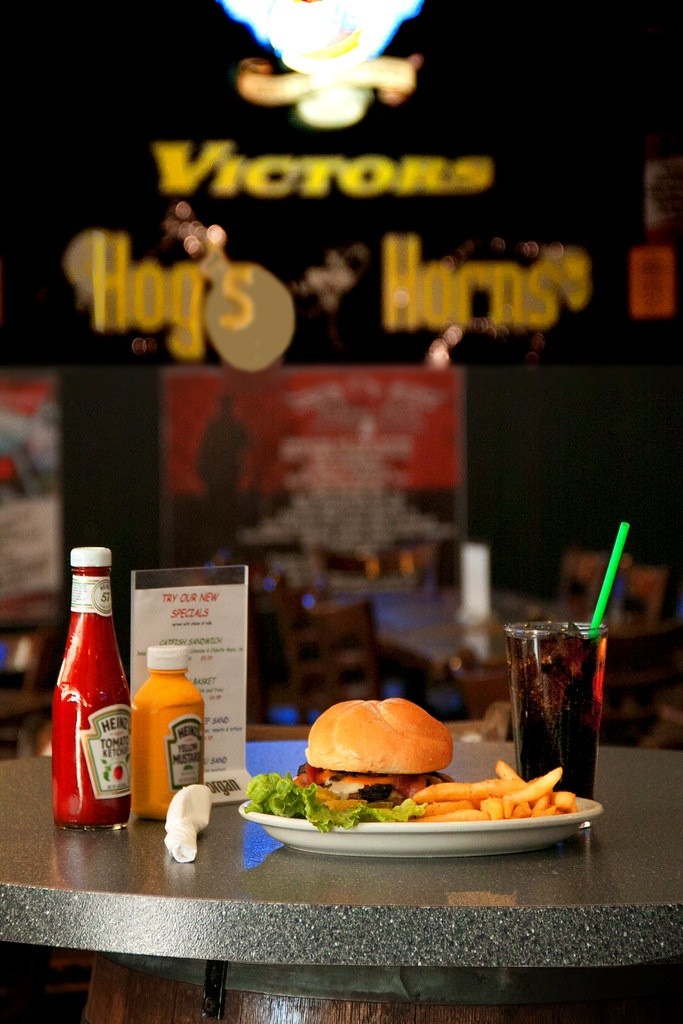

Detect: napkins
164;784;212;862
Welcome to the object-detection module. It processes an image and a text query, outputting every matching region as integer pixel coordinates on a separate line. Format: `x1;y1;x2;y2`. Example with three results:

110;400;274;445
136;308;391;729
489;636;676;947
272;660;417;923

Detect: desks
0;740;683;1024
351;590;675;685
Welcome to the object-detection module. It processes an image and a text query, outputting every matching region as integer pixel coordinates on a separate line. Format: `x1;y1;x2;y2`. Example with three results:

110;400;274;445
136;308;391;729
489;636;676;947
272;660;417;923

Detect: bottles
131;643;205;820
50;546;132;829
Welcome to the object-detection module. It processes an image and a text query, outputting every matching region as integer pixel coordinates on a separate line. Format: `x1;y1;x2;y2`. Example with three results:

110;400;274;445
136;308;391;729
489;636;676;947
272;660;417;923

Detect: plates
238;797;604;858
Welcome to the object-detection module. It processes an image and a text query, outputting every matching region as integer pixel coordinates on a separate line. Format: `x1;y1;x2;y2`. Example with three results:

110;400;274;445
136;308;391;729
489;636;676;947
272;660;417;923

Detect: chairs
561;555;683;745
257;575;439;724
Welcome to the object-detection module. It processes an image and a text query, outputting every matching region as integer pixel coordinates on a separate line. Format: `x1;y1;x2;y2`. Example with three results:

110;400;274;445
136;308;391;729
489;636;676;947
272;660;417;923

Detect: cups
504;620;608;829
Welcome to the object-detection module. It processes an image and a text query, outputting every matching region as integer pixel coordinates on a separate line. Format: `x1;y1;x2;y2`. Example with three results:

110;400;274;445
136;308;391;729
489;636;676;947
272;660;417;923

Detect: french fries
408;759;579;822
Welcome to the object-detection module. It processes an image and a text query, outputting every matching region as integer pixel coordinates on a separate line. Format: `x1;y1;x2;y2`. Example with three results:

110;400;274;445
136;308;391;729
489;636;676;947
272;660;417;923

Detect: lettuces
242;770;426;834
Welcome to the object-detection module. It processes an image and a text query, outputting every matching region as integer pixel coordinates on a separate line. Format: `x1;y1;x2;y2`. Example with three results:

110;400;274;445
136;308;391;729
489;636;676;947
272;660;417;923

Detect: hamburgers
296;698;454;812
